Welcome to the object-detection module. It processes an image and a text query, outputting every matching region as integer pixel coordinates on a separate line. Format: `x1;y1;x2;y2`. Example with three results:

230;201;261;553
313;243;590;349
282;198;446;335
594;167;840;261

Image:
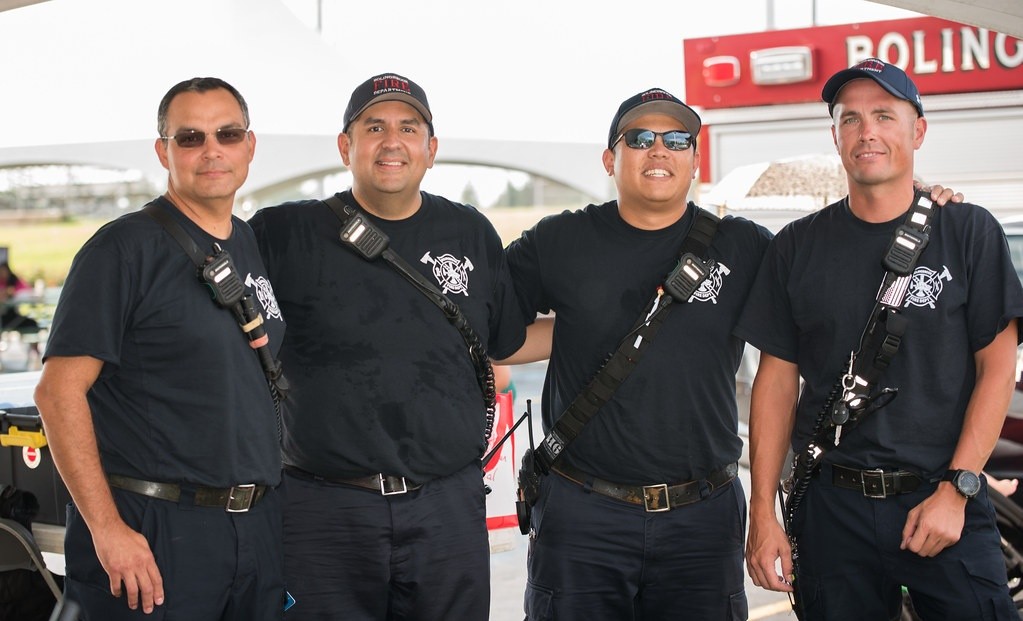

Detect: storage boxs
0;407;69;526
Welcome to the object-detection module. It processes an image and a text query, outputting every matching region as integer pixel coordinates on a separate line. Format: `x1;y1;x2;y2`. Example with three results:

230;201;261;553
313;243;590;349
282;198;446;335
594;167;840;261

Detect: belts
107;474;268;513
285;465;424;497
792;455;921;498
550;458;738;513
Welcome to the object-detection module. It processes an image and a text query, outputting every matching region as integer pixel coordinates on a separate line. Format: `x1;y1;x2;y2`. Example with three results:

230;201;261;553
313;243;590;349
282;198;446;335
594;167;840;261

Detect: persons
728;58;1023;621
0;260;23;317
503;86;965;621
246;73;556;621
33;76;288;621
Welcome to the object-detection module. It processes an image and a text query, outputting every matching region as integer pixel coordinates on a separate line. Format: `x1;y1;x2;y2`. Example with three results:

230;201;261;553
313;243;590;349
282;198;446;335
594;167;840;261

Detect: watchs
928;469;981;499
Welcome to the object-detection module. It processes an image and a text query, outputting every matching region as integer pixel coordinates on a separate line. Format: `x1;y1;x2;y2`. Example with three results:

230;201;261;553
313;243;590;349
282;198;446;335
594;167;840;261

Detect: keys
830;399;849;446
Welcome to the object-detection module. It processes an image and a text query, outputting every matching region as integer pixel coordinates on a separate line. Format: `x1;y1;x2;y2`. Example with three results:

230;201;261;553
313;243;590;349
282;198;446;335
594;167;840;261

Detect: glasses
161;128;250;148
610;128;695;151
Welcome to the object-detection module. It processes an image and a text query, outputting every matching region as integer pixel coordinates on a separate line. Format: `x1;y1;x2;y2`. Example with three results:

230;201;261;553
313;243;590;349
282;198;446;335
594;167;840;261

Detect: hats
608;88;701;154
822;58;924;117
343;73;434;136
